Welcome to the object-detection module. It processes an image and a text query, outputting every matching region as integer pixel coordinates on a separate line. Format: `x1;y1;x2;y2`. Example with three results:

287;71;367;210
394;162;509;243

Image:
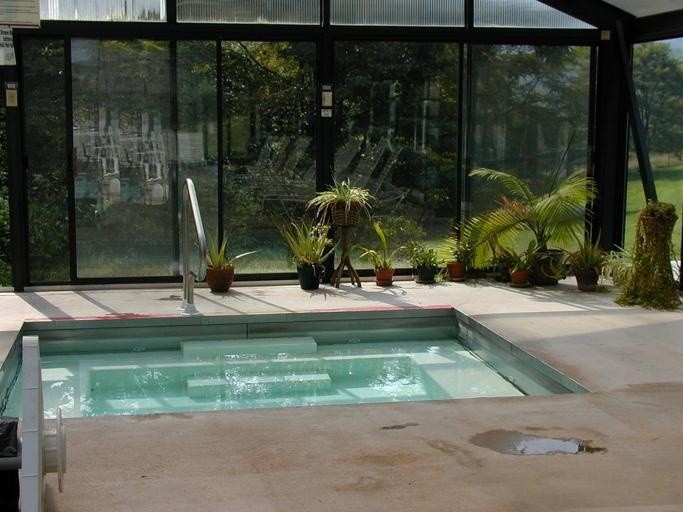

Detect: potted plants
197;235;260;293
354;220;406;286
491;240;541;288
607;194;681;310
560;220;605;292
408;245;443;284
449;166;606;287
305;175;376;225
273;216;340;291
446;229;477;283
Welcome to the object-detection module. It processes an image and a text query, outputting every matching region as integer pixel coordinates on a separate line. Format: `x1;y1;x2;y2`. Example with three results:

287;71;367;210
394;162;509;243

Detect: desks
331;223;363;288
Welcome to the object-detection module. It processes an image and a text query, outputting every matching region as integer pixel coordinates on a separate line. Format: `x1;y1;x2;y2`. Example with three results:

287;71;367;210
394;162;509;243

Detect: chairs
72;120;170;220
223;124;423;228
